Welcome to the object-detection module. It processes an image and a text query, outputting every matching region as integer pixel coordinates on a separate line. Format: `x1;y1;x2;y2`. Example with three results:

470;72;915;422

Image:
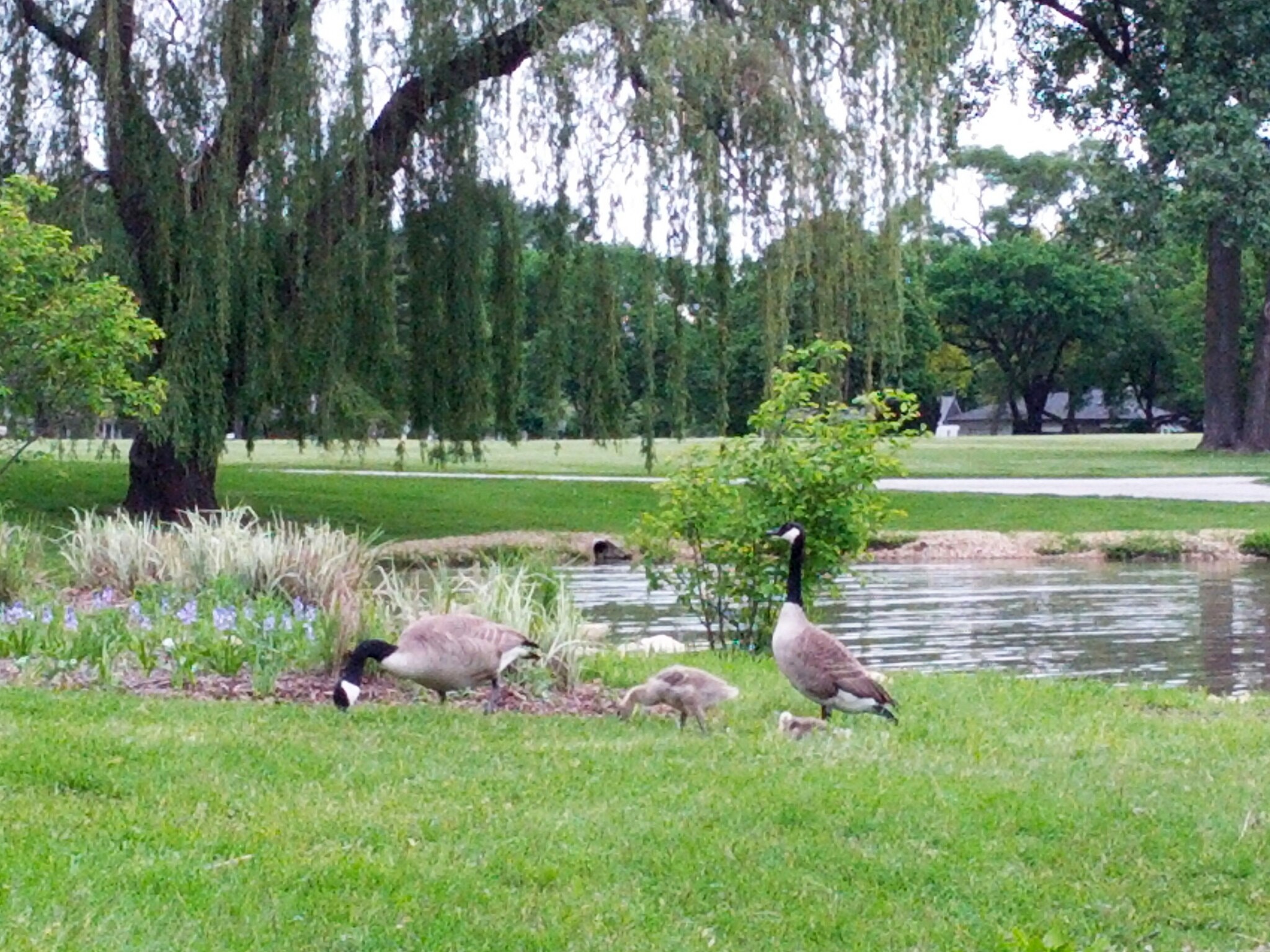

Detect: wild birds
334;613;541;712
766;522;898;723
778;712;831;742
619;667;736;733
